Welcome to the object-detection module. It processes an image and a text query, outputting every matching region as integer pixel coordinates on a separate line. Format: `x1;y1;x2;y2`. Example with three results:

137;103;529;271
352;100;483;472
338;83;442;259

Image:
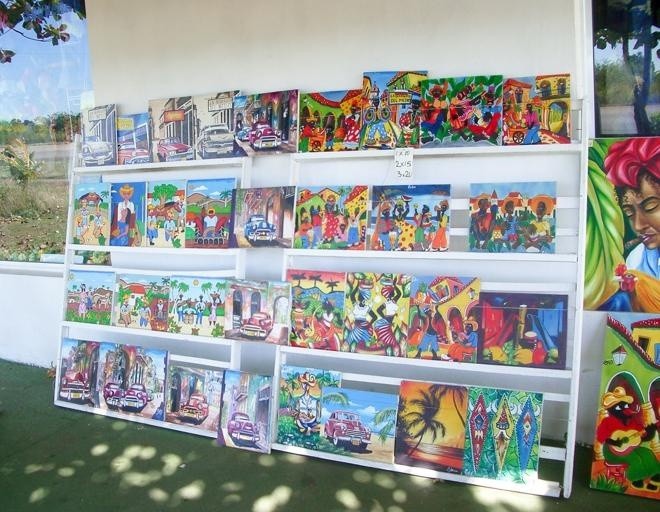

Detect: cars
79;117;285;169
238;311;274;340
244;213;278;244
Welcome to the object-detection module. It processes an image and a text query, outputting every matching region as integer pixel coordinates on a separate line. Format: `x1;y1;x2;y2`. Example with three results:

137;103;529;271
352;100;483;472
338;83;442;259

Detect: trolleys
506;128;530;144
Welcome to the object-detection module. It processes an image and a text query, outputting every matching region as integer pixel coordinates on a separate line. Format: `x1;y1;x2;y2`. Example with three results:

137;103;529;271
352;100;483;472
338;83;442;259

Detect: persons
604;138;660;311
